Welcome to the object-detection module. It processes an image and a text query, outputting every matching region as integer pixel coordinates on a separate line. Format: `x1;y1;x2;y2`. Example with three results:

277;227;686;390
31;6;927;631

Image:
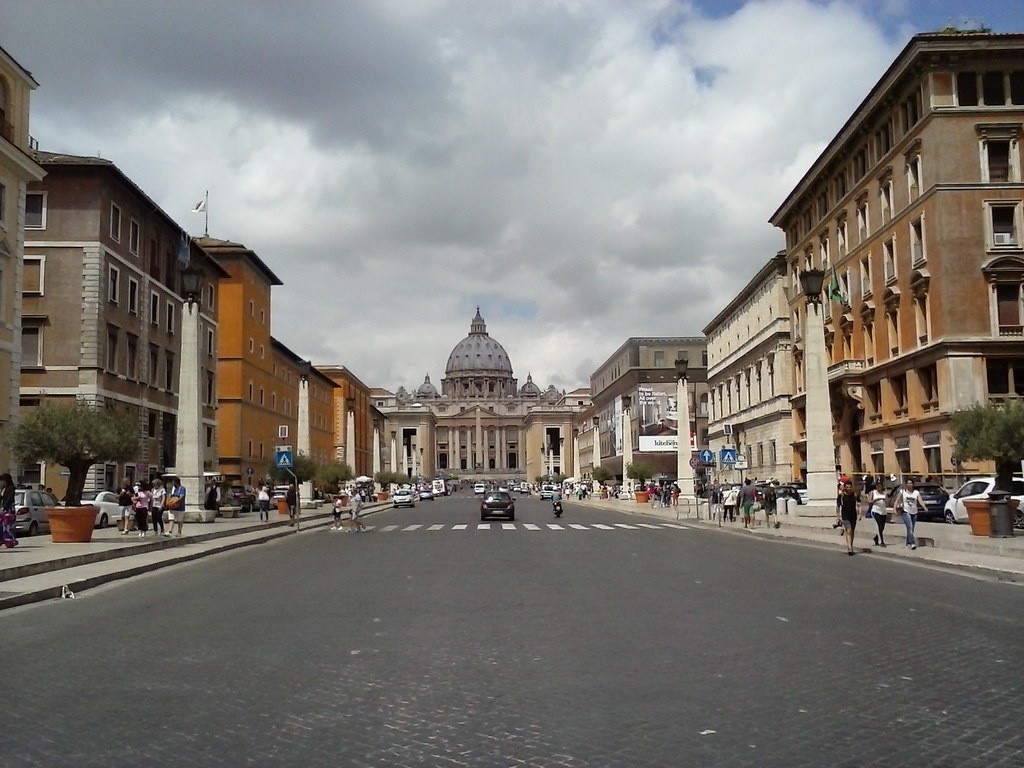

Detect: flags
192;198;206;212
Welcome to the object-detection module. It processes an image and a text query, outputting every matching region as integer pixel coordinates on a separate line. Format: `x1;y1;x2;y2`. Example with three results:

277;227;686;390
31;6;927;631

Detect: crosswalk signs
277;450;292;468
721;448;736;465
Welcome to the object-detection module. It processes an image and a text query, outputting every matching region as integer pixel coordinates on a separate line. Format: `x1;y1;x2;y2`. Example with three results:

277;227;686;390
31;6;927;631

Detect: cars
392;489;416;508
419;489;436;501
55;488;133;530
886;480;950;521
539;483;557;500
11;487;64;536
701;479;807;512
474;483;487;493
496;482;534;494
943;477;1024;528
220;483;288;515
481;490;518;522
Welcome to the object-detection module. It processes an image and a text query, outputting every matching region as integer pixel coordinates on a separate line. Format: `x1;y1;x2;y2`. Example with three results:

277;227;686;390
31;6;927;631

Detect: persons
205;480;221;511
868;481;891;547
645;482;680;508
333;485;374;531
550;487;562;512
839;481;862;554
115;477;186;537
26;484;52;492
525;481;559;495
838;474;849;490
380;483;433;494
246;481;272;522
286;484;296;519
894;480;928;550
562;482;622;501
865;471;874;494
0;473;18;545
708;474;779;528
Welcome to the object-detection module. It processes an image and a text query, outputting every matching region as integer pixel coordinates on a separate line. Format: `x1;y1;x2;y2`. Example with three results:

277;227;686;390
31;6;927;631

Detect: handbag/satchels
896;489;903;513
166;495;180;509
865;491;874;518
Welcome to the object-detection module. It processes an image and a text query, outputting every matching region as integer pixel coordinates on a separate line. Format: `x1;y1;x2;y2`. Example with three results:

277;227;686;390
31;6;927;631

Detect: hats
674;481;677;484
766;479;771;484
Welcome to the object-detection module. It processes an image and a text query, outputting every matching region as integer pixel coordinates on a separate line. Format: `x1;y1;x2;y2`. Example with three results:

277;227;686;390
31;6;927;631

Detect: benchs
315;500;326;507
886;507;905;523
219;506;243;518
617;493;636;500
110;514;135;531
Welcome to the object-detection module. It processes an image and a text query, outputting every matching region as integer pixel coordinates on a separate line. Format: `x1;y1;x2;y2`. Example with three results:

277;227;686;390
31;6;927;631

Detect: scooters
553;501;564;518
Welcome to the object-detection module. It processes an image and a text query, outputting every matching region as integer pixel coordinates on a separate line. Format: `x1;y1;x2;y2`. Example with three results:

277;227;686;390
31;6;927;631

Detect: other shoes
338;526;343;530
910;545;915;550
175;533;181;538
874;534;879;545
881;543;886;548
164;531;172;537
848;550;855;556
331;527;337;530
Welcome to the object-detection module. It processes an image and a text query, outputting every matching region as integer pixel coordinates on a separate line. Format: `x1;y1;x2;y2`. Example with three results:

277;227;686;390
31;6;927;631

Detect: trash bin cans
988;490;1014;537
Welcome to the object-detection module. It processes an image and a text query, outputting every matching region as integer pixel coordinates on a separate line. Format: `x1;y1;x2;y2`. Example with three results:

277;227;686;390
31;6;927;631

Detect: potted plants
591;465;616;497
374;471;393;500
265;452;315;513
317;459;359;507
945;393;1024;536
625;460;657;503
1;389;143;543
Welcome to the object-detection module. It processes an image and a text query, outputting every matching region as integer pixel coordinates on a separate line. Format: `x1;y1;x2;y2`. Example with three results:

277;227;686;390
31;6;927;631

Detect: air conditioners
993;232;1010;243
